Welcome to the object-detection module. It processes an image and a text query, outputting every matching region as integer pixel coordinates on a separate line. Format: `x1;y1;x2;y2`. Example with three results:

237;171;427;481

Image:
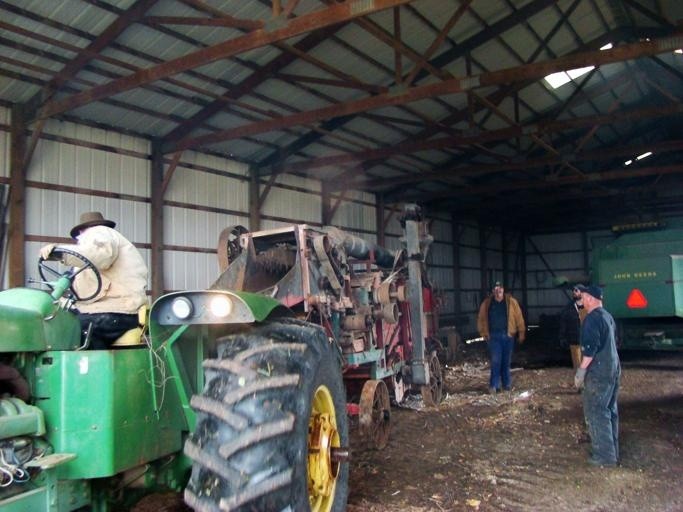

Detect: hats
573;284;586;291
491;281;504;288
585;286;603;299
71;212;116;236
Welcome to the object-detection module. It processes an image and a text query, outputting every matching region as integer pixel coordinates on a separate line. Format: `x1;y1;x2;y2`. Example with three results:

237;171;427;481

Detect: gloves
37;244;65;262
574;367;587;388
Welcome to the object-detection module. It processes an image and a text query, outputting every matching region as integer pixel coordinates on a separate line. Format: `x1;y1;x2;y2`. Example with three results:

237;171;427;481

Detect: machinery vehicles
0;223;362;512
544;221;682;366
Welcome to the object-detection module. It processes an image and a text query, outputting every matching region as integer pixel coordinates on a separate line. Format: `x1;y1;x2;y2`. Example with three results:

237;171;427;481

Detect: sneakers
585;454;617;469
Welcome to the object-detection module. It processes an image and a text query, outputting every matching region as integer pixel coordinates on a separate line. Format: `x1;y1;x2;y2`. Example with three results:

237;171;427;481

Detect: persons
477;281;526;393
560;283;586;392
575;283;620;468
40;212;149;352
